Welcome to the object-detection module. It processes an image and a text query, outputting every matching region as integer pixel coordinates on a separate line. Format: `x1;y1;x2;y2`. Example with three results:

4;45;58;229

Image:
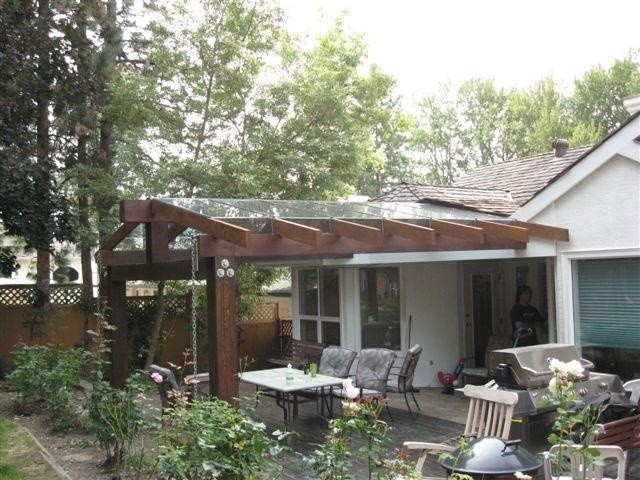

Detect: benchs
265;339;325;404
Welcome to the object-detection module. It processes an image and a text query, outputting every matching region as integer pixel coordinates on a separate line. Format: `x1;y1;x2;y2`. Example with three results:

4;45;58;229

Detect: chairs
403;384;518;479
544;443;626;480
295;344;423;428
149;364;182;420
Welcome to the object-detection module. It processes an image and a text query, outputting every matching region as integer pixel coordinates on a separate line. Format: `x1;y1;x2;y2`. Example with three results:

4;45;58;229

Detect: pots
438;434;544;480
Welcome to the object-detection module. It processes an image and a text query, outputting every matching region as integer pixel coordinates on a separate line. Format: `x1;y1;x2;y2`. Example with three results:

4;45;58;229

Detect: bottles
285;363;295;383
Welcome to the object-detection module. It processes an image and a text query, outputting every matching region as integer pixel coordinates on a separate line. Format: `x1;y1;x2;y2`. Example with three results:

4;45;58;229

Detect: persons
509;283;549;347
53;260;79;280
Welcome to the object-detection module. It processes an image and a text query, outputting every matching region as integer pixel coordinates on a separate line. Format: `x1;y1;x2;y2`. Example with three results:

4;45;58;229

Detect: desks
238;368;345;444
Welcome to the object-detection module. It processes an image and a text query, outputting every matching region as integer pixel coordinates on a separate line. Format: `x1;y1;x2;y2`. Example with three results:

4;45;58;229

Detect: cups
309;363;317;377
